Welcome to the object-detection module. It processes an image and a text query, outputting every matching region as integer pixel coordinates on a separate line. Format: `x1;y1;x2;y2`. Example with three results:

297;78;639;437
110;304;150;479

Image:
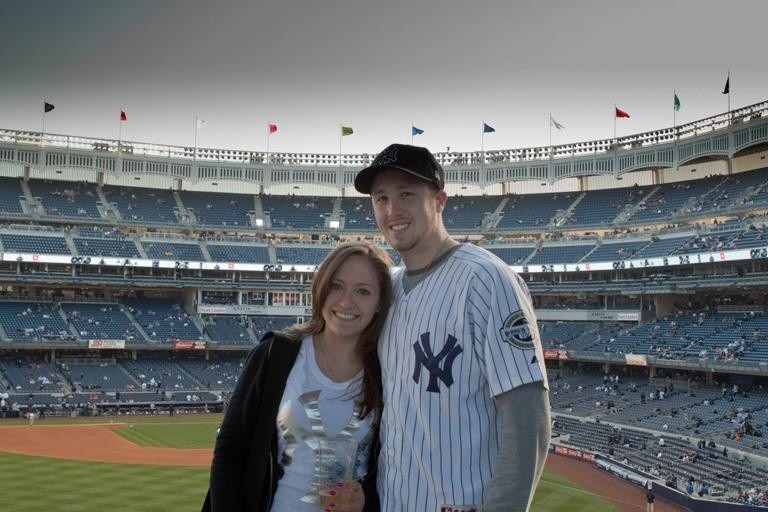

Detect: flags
44;103;54;113
412;128;424;136
120;111;126;121
196;117;207;128
674;95;682;113
483;124;495;134
721;78;729;94
552;117;564;131
269;123;276;133
341;127;354;136
616;108;630;119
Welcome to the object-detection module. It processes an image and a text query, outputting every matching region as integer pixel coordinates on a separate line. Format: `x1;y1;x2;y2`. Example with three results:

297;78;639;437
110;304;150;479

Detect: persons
196;238;391;511
350;142;552;512
26;404;36;427
350;189;554;346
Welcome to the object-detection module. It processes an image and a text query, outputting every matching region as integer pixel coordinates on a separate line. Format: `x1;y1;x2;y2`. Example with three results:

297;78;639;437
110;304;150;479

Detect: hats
354;144;445;194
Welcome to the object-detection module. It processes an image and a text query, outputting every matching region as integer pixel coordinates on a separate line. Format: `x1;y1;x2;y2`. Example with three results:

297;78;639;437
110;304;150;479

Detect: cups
317;436;359;511
